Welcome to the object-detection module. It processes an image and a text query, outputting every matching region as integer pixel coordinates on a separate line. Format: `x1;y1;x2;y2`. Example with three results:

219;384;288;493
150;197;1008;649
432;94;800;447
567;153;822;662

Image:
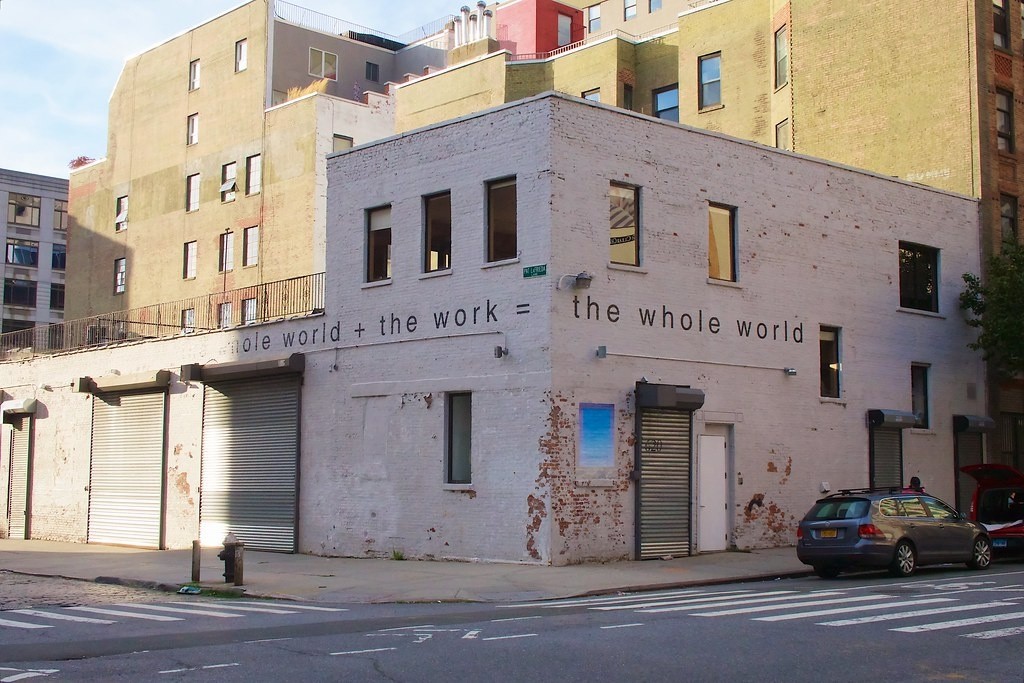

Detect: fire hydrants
216;532;239;583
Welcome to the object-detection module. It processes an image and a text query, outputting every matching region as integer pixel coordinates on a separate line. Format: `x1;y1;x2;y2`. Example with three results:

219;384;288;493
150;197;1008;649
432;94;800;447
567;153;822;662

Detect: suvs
797;486;994;579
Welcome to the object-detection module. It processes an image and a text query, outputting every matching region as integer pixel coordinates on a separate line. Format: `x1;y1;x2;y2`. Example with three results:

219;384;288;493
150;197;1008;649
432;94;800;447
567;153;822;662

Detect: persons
1008;490;1017;506
902;477;926;494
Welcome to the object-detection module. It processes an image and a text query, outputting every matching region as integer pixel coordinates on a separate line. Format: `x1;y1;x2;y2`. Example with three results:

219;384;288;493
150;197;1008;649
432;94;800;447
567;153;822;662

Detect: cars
960;464;1024;553
